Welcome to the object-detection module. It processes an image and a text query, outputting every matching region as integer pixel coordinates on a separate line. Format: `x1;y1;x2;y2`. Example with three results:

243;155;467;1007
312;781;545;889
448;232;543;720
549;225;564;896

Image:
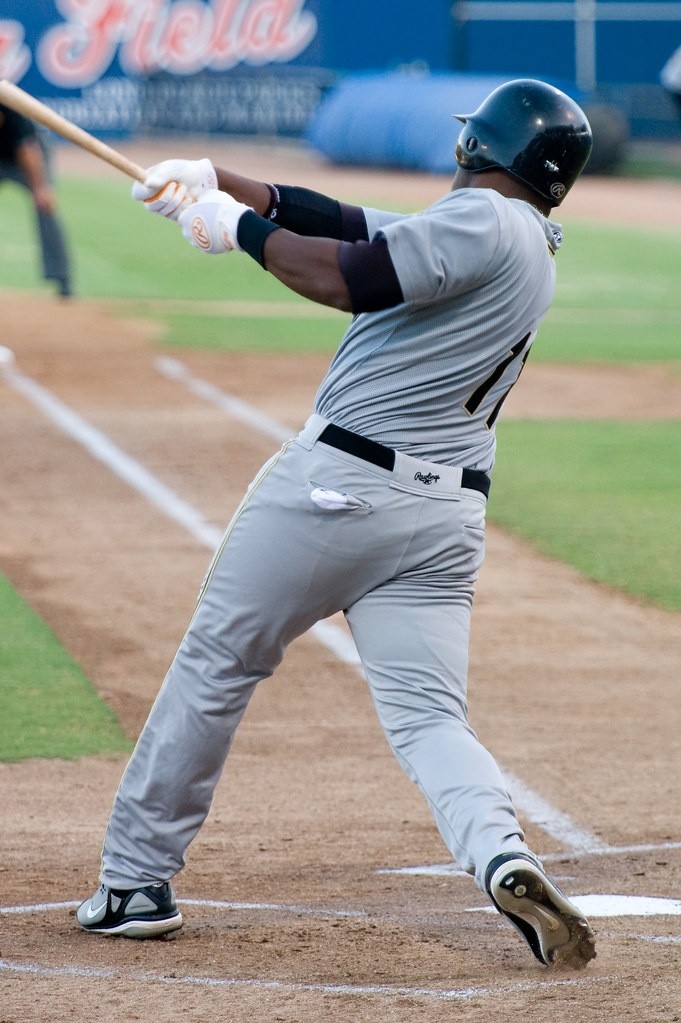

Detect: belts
317;423;491;500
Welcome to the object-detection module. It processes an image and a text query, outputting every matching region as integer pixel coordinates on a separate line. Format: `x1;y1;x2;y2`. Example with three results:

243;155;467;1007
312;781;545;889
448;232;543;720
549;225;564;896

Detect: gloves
178;188;256;254
131;157;219;222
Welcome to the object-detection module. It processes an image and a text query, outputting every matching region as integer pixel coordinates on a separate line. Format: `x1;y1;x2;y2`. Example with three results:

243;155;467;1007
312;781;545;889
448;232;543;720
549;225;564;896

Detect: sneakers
484;852;597;971
76;882;182;938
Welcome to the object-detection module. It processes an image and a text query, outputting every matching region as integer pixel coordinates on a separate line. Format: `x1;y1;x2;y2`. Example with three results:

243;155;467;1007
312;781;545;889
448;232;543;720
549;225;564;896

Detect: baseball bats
0;77;150;185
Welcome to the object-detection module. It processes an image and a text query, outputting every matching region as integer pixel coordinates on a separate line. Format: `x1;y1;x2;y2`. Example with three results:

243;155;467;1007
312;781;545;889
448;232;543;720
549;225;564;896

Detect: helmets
451;79;593;207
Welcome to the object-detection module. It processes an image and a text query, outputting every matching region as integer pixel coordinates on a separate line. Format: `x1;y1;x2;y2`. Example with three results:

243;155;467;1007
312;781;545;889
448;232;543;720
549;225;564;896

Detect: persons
0;102;74;298
73;78;597;972
